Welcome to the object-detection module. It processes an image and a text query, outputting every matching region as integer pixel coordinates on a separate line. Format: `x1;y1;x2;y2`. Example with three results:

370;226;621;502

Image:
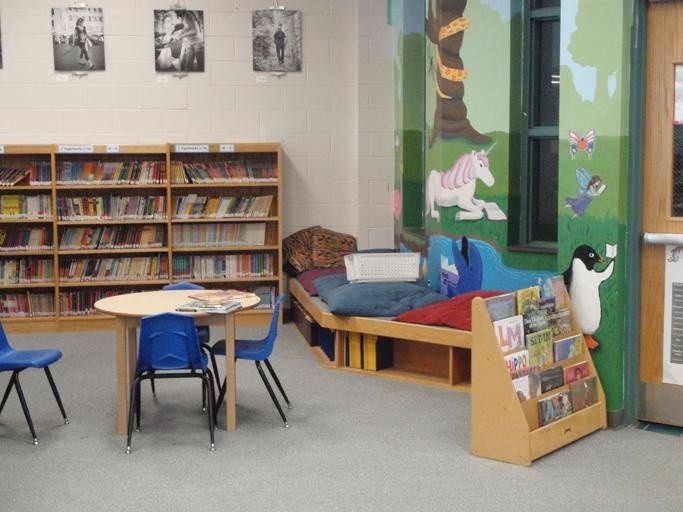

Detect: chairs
125;312;219;455
159;281;222;414
0;321;70;446
210;292;293;429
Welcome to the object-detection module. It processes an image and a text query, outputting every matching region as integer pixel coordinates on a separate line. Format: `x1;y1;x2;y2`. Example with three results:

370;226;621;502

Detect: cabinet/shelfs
0;141;284;335
469;279;608;466
286;276;472;394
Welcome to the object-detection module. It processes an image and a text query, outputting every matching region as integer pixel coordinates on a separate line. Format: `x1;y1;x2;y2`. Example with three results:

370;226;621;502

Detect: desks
93;289;261;435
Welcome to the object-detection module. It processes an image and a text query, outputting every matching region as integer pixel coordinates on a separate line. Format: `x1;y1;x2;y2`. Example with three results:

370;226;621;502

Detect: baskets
345;252;421;282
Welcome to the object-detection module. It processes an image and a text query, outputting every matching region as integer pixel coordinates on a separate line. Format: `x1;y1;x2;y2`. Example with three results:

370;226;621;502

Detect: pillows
295;269;346;296
282;225;321;273
390;289;511;331
283;261;297;278
312;273;349;302
311;227;358;269
323;283;450;317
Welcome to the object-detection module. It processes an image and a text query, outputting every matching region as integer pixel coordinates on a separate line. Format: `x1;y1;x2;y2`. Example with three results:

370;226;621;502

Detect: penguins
560;244;615;351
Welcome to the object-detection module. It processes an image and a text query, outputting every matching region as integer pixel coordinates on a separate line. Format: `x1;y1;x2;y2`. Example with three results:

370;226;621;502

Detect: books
171;192;274;219
55;158;167;185
1;225;54;251
171;223;266;248
59;223;165;250
1;289;55;313
59;287;162;313
188;288;256;301
56;192;168;220
1;193;51;219
171;253;274;280
211;284;275;310
1;159;52;186
2;257;55;284
175;301;241;313
170;157;275;184
482;275;600;427
59;252;169;281
